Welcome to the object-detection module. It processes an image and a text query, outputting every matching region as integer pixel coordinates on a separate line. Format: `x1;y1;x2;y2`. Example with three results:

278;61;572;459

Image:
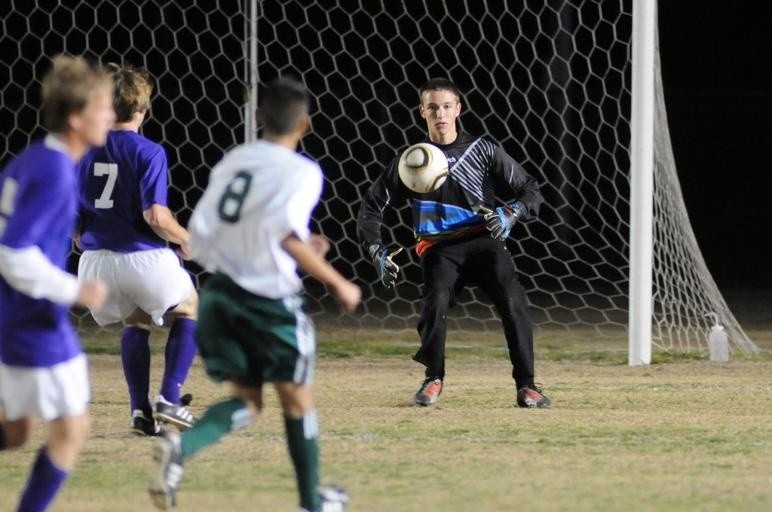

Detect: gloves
368;241;403;289
479;201;525;242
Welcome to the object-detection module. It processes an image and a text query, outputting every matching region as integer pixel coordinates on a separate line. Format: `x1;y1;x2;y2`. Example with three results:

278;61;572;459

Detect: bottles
704;313;729;364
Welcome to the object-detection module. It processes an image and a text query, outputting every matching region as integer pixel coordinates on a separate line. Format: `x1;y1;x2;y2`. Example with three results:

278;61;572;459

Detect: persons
0;56;119;512
356;77;550;410
147;76;362;512
77;66;202;437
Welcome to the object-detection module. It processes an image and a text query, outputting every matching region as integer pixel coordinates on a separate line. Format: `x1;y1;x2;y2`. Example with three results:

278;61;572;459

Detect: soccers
398;141;449;193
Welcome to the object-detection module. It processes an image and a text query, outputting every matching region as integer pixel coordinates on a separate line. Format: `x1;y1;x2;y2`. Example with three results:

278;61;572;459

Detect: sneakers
517;387;550;408
416;379;442;405
298;485;349;512
131;394;197;509
